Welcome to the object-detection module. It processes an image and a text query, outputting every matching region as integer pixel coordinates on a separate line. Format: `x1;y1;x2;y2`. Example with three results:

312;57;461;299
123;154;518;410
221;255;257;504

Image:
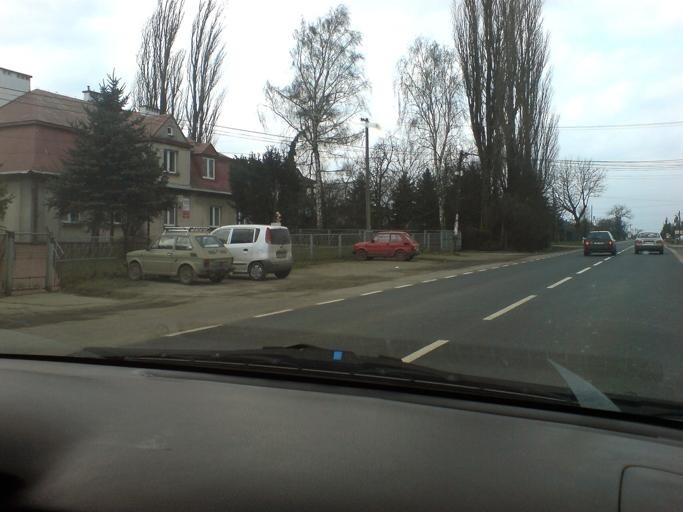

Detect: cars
583;228;617;255
131;235;234;285
633;231;664;254
352;230;420;262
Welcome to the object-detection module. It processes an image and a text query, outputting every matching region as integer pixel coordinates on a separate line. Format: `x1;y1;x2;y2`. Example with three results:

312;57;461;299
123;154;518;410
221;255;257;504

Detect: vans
188;224;293;281
